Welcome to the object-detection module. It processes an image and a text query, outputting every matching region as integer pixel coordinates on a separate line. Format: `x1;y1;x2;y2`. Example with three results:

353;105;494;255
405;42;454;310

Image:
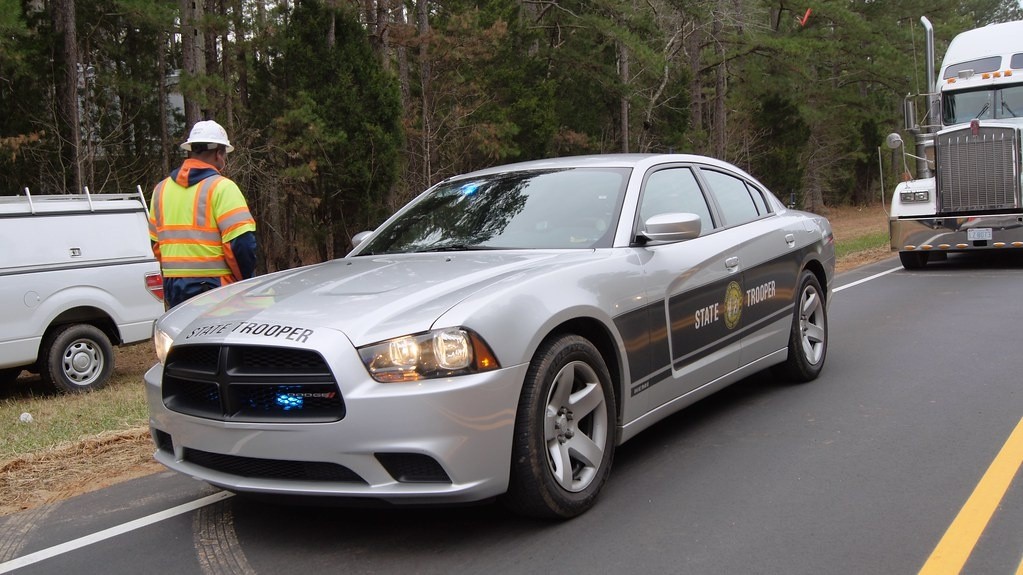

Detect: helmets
180;120;234;153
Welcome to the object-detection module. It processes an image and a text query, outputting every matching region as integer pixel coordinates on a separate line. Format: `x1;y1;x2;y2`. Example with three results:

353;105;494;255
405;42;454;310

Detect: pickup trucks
0;186;166;398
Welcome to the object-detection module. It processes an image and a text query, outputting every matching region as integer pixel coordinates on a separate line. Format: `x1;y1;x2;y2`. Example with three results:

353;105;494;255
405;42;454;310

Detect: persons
148;120;258;312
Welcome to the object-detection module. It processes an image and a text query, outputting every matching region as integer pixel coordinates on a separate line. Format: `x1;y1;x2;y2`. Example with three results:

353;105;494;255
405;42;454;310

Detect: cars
142;152;841;527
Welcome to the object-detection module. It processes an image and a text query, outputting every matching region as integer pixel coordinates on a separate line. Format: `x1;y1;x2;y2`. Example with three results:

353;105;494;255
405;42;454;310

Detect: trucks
878;15;1022;270
